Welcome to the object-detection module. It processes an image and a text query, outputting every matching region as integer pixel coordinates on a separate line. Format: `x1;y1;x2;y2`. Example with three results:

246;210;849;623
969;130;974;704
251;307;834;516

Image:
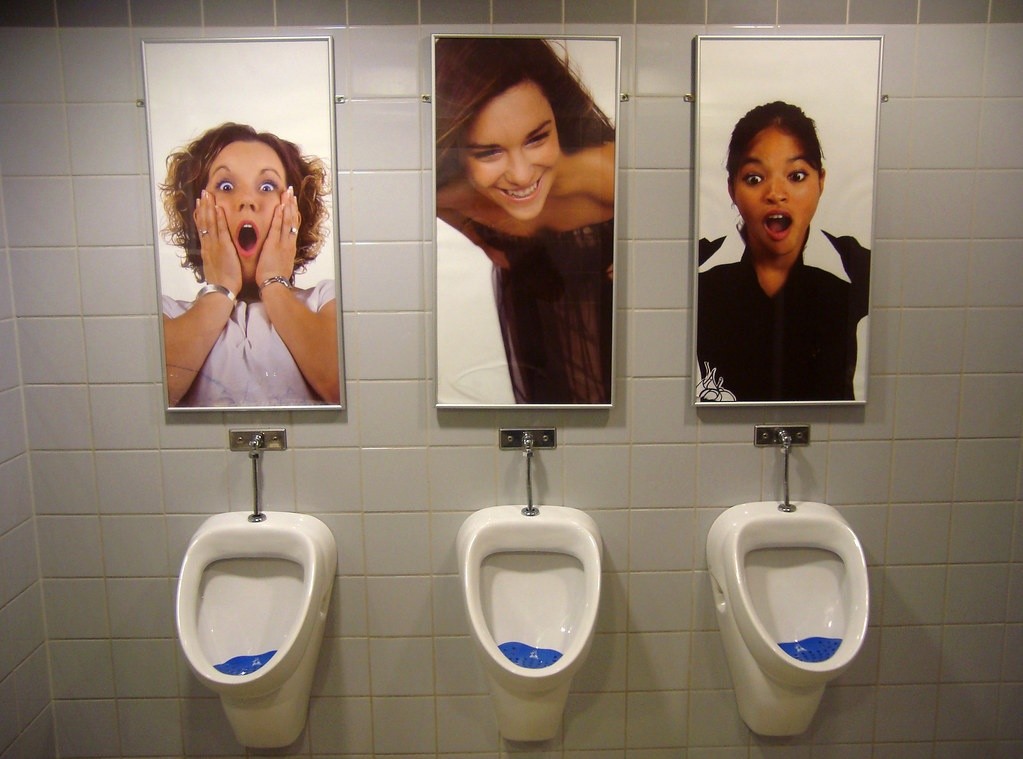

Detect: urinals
456;505;604;744
175;511;338;748
707;502;869;736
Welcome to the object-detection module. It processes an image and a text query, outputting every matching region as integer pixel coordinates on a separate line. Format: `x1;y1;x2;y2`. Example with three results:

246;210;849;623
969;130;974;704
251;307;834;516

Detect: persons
435;36;616;405
159;122;341;407
698;101;870;403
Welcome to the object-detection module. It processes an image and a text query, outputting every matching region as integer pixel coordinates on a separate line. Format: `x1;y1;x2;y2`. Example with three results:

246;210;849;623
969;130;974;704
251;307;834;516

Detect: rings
291;228;298;234
201;230;207;236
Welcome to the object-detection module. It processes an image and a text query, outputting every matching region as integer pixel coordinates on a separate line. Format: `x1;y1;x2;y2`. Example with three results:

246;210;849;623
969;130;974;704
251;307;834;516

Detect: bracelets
258;276;292;292
196;284;237;308
